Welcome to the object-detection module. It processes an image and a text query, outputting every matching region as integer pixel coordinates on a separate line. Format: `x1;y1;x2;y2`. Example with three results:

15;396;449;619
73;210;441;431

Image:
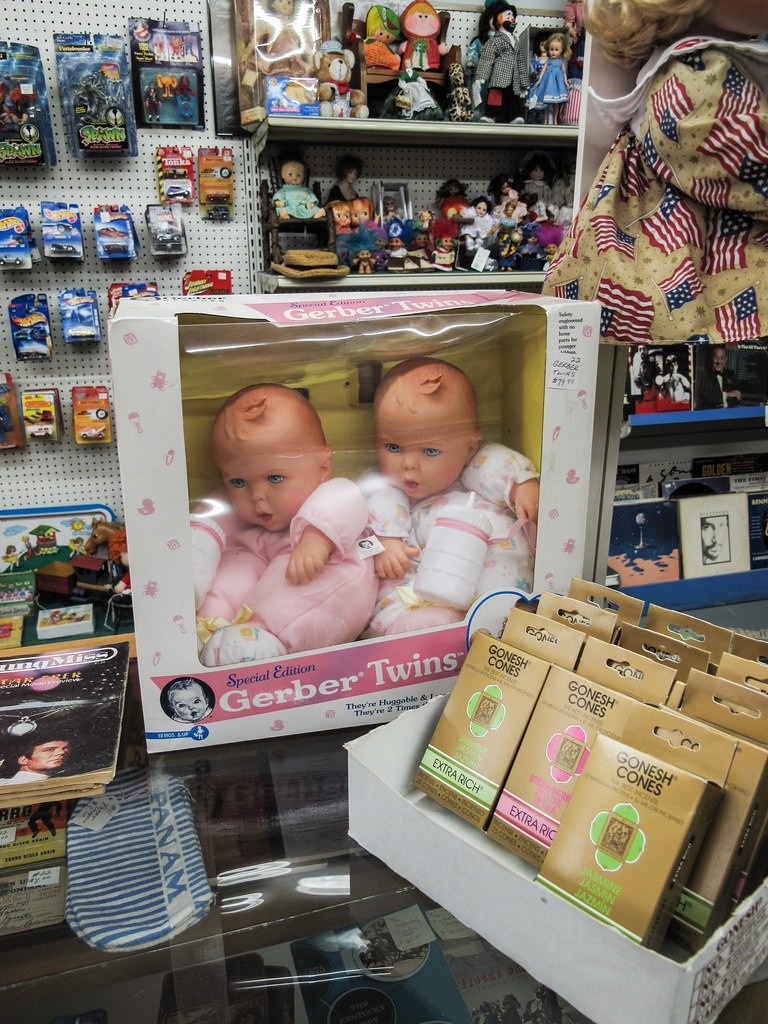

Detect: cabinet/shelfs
243;0;768;609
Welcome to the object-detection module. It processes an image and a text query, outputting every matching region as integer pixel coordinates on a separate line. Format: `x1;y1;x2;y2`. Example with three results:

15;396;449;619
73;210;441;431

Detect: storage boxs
342;577;768;1024
